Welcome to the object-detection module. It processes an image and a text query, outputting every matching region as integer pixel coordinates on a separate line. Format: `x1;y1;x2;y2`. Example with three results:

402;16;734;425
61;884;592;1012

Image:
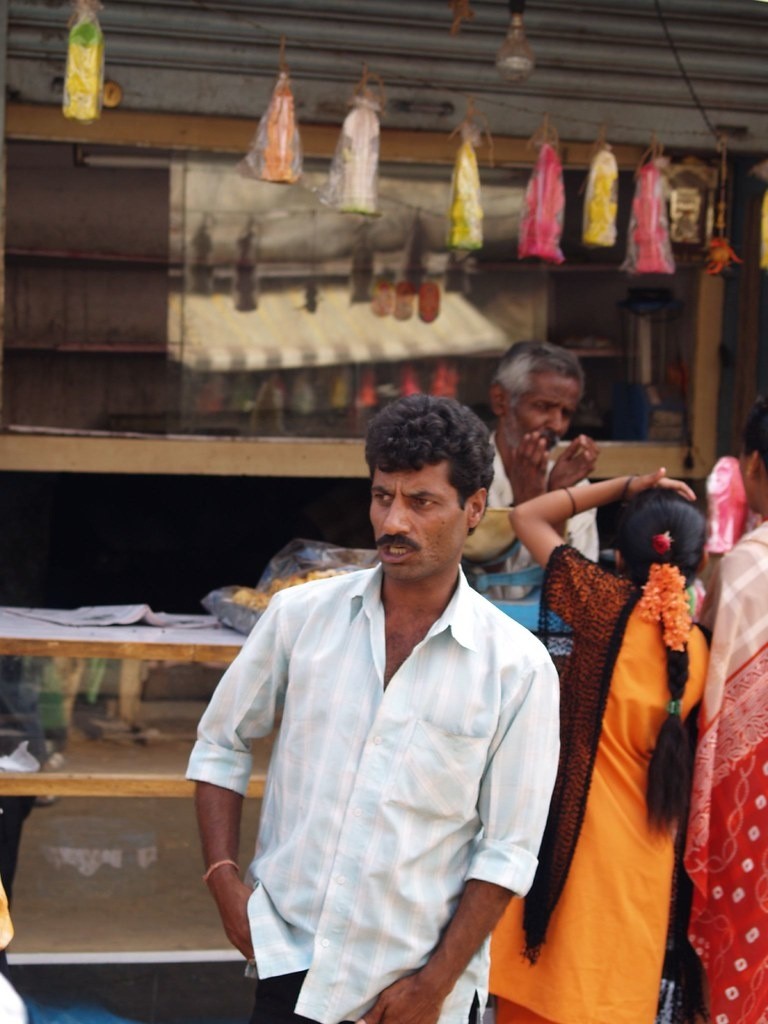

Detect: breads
230;568;350;611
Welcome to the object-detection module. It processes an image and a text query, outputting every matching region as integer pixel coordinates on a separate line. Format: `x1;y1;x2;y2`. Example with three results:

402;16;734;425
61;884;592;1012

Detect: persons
185;394;560;1024
684;398;768;1024
489;467;713;1024
463;339;598;603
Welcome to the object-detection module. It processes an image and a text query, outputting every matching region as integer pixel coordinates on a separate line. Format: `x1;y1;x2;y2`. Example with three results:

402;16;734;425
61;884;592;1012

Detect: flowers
637;562;693;652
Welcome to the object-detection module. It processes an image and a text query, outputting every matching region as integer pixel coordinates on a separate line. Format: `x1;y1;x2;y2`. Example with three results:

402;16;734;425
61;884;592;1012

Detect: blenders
610;285;689;441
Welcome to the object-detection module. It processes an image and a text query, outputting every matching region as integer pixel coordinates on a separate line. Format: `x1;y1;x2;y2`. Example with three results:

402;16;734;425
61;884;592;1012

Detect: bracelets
202;860;240;881
560;486;576;517
622;474;640;499
